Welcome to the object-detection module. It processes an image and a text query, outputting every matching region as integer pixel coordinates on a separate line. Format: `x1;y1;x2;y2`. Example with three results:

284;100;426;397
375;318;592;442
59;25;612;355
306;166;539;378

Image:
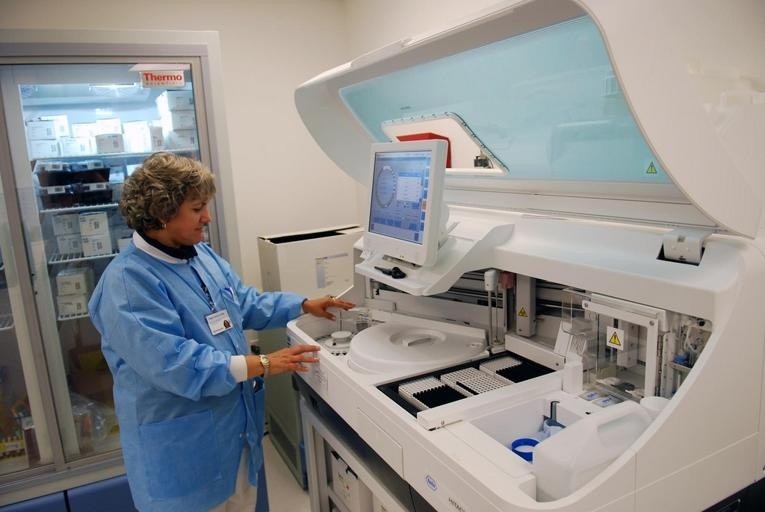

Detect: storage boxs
256;224;364;290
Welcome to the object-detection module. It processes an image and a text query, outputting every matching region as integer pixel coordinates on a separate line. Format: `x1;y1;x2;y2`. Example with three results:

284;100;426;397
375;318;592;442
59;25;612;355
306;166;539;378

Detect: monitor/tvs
364;139;448;267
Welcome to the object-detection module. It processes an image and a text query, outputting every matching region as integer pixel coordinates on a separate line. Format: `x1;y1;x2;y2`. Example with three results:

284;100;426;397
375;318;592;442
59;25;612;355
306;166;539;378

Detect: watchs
260;354;270;379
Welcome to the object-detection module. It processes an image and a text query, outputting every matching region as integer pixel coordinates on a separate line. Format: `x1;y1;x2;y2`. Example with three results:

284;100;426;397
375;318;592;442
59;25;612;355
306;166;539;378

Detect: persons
86;151;355;512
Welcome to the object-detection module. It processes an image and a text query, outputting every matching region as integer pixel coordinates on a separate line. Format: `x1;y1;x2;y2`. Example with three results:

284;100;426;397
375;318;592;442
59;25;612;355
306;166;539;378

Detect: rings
329;296;335;305
